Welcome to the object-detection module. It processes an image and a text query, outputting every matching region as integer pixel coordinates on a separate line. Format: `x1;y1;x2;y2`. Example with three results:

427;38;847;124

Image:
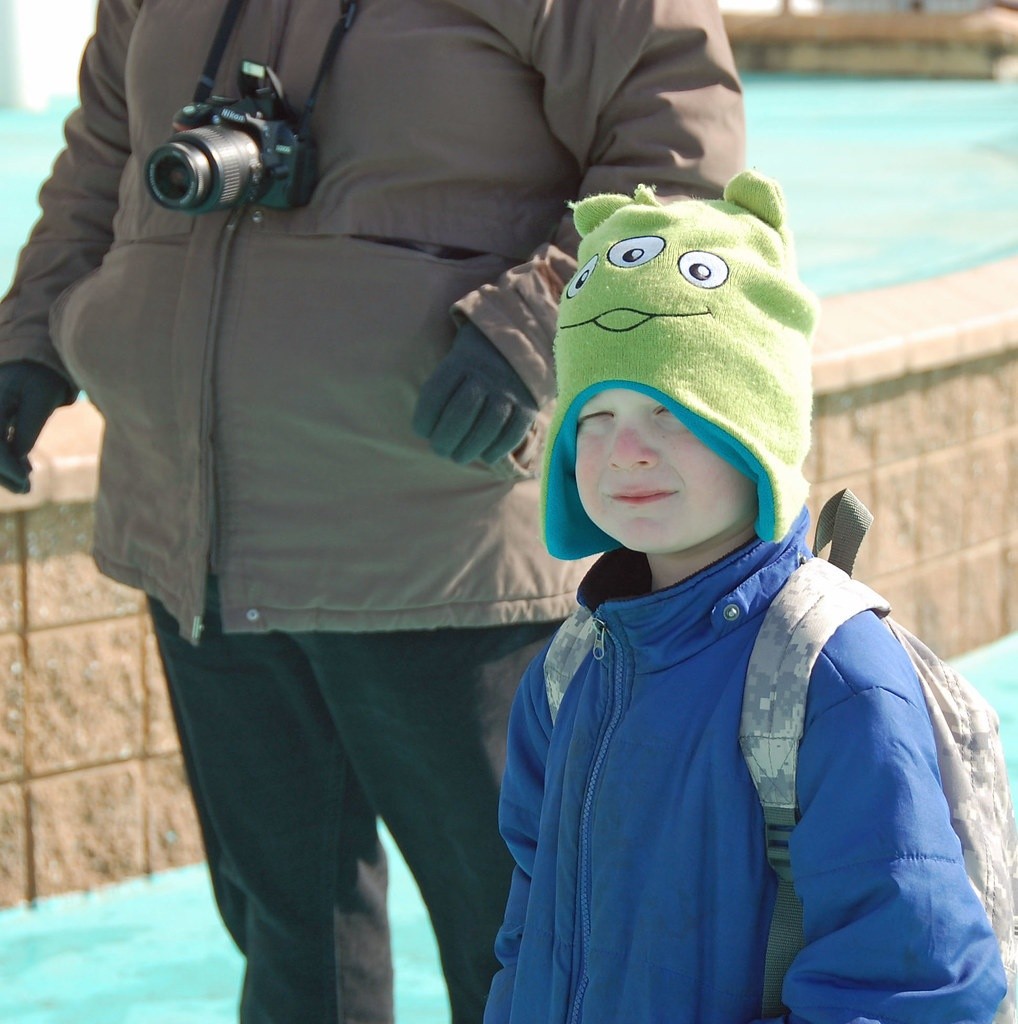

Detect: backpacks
546;486;1017;1024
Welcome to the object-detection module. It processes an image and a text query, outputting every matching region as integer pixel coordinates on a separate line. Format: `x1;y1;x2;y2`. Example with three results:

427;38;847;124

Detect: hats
536;168;816;555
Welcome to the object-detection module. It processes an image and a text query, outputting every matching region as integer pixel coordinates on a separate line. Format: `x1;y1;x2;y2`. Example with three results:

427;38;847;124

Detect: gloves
0;359;76;498
417;311;537;464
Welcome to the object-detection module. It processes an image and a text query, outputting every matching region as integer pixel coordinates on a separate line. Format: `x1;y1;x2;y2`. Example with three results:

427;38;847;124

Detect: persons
483;171;1008;1024
0;0;744;1023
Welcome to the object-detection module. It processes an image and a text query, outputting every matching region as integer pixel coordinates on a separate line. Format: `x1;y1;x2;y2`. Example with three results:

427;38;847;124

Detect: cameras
145;62;318;214
